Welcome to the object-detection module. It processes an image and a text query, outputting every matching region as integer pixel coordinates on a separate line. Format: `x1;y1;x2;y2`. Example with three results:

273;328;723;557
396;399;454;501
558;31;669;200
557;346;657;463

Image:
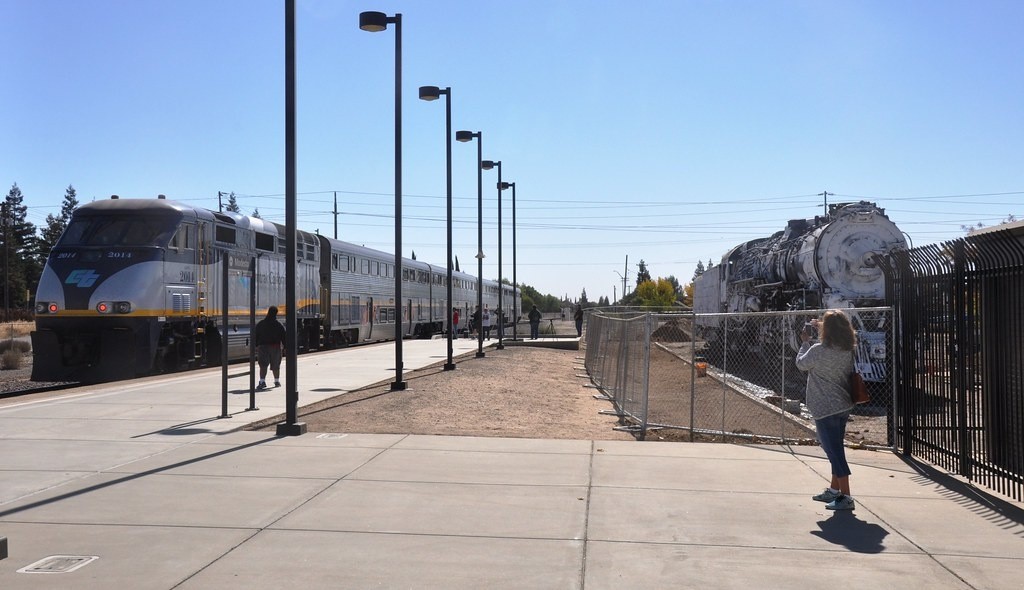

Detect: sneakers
824;493;855;510
811;488;840;503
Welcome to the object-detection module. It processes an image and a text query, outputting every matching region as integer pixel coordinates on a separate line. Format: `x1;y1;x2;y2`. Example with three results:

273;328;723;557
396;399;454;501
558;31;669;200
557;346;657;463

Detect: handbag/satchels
852;373;870;405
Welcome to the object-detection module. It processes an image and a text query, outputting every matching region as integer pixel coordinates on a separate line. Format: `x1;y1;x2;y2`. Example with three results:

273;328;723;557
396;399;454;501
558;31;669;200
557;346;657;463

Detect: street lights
358;9;410;391
480;159;501;349
456;129;488;357
418;83;455;369
496;181;518;342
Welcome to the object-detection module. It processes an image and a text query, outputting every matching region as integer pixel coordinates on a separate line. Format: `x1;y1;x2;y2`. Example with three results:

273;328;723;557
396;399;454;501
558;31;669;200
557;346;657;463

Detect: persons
452;307;459;339
482;307;491;341
494;308;505;339
252;306;286;389
796;311;859;510
528;304;542;340
574;304;584;336
471;306;480;339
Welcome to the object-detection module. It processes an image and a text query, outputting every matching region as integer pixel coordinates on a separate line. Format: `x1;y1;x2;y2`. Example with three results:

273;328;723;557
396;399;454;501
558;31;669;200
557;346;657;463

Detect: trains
690;198;912;393
28;193;523;381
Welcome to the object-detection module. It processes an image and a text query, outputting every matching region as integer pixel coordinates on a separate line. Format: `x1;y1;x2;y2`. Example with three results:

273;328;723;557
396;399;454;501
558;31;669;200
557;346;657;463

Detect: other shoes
274;382;281;387
257;381;267;389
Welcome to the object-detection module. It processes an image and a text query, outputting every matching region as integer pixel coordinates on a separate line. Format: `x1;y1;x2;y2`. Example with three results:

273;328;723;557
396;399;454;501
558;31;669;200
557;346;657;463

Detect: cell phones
805;323;812;338
